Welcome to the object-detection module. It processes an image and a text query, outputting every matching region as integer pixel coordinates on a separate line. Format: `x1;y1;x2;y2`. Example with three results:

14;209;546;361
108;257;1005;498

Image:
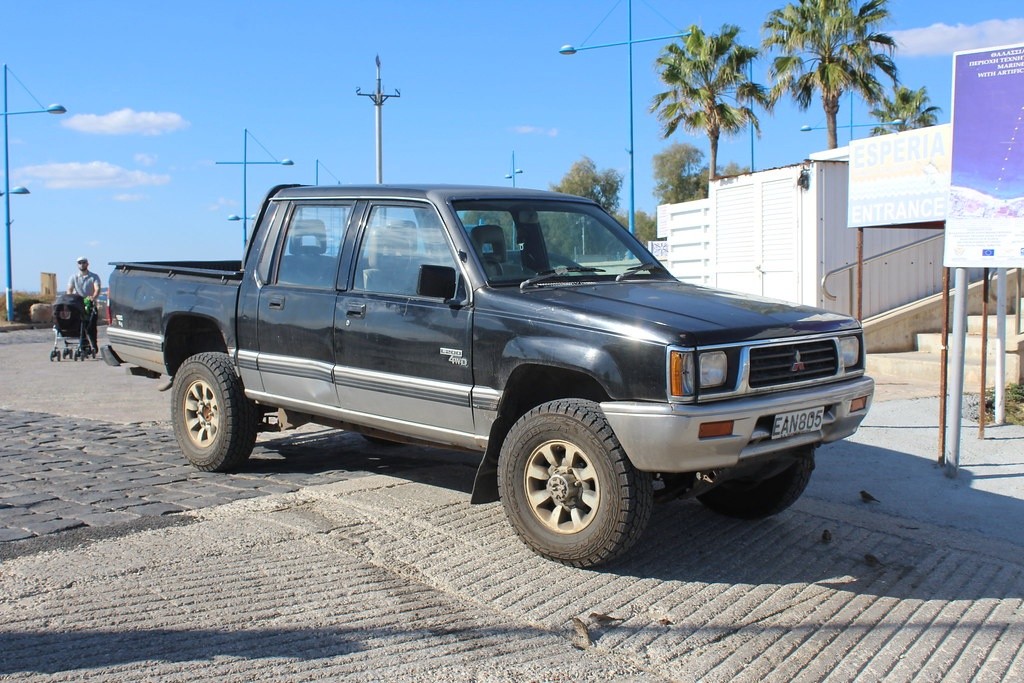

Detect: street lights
800;89;904;142
0;63;66;324
504;150;523;188
558;0;691;261
215;128;294;250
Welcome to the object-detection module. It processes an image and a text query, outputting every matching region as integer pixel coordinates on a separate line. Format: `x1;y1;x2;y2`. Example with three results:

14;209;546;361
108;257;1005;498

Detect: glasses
78;262;87;264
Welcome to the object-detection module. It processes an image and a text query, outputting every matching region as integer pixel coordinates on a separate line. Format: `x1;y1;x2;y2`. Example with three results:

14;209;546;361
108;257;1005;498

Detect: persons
67;257;101;354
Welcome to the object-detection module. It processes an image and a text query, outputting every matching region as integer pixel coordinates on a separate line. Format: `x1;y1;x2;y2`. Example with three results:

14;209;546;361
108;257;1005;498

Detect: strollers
49;295;97;362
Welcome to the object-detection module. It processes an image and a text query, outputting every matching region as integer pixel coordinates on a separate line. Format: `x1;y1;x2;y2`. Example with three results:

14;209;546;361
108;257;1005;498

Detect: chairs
385;220;418;260
469;224;515;280
280;220;337;287
362;227;419;294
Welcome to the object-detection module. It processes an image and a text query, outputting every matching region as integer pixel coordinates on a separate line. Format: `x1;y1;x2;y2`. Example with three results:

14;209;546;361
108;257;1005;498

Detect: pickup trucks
102;182;874;570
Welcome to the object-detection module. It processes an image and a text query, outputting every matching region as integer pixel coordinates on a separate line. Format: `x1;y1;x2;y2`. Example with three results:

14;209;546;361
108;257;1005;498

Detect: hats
76;257;87;262
